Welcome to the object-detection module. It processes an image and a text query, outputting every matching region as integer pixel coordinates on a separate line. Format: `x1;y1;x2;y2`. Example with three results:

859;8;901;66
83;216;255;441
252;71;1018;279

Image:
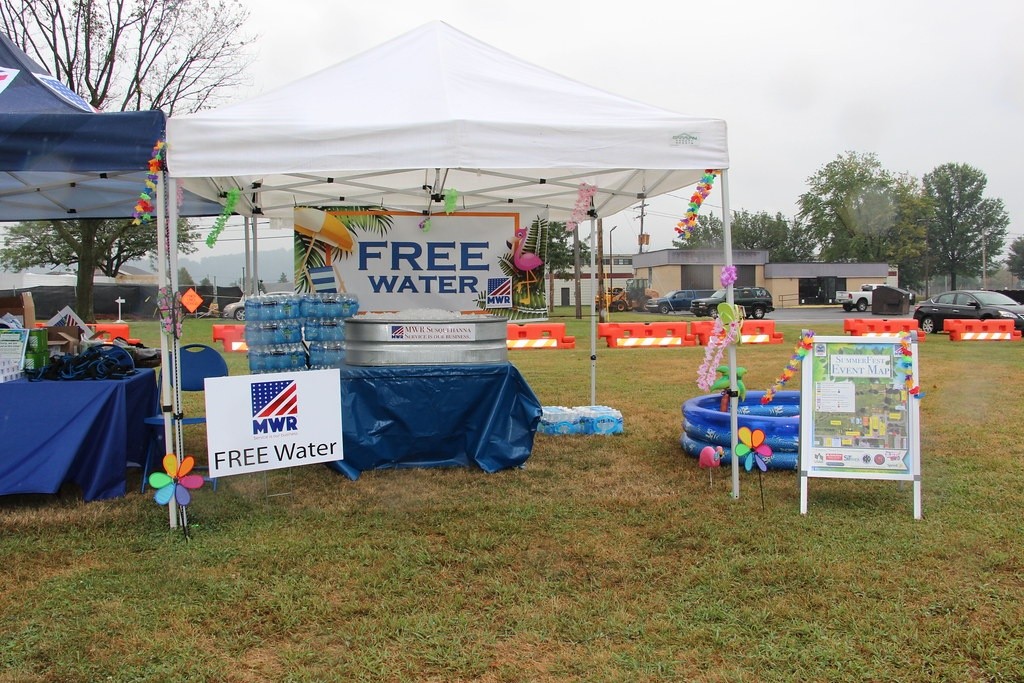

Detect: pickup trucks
836;283;913;312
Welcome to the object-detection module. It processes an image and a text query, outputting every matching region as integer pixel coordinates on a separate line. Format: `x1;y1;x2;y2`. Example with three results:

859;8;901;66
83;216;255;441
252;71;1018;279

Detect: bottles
244;293;359;373
536;404;624;436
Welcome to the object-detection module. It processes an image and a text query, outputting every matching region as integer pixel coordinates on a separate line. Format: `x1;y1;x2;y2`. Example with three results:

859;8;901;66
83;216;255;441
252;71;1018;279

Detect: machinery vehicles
595;279;660;313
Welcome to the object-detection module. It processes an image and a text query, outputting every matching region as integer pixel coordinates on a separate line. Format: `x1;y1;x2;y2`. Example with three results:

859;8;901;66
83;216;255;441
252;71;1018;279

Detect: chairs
88;343;135;370
142;344;229;495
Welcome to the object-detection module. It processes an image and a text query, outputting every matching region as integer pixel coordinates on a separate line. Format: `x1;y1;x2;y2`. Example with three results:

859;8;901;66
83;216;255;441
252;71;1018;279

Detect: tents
166;17;741;527
0;29;177;528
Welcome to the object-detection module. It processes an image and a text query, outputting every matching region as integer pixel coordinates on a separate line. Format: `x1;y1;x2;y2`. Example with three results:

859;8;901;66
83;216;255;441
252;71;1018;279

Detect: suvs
647;290;716;314
691;287;776;320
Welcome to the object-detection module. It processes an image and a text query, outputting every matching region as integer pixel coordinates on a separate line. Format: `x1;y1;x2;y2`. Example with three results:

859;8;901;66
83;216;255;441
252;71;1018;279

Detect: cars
223;292;295;322
913;289;1024;337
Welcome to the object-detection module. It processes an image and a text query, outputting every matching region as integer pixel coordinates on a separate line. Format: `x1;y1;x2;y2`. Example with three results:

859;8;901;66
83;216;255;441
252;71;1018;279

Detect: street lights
609;225;617;312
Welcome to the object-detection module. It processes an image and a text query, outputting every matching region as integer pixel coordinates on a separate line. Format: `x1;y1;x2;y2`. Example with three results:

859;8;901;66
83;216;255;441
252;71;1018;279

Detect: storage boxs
0;292;79;357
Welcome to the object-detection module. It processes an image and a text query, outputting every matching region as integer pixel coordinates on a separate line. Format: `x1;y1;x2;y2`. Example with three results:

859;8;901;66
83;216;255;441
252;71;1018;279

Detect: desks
0;368;158;503
318;364;544;478
